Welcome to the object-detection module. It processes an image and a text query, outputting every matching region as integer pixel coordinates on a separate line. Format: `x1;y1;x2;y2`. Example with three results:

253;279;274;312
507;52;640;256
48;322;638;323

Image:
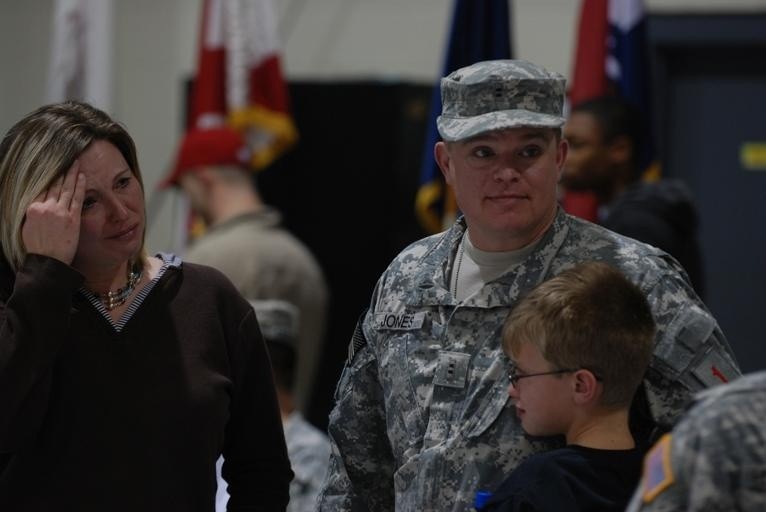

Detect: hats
249;298;298;345
437;60;567;142
156;126;242;185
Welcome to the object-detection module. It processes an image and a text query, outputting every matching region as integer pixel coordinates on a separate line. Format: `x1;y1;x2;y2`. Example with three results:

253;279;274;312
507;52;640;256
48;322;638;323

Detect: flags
47;0;112;118
559;0;653;223
415;1;513;234
184;0;290;256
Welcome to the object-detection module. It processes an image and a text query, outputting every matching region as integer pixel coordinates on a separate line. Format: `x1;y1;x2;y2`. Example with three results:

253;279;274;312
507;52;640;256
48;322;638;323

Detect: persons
476;260;658;510
314;58;745;512
215;297;332;512
0;99;296;512
558;92;707;307
158;111;330;415
626;369;766;511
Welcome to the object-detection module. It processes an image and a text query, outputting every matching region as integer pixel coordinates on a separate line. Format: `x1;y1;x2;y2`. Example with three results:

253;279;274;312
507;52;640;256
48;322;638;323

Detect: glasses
504;366;600;387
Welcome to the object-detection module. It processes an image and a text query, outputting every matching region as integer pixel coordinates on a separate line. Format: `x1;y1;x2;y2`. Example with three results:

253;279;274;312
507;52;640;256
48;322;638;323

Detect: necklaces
93;252;144;311
